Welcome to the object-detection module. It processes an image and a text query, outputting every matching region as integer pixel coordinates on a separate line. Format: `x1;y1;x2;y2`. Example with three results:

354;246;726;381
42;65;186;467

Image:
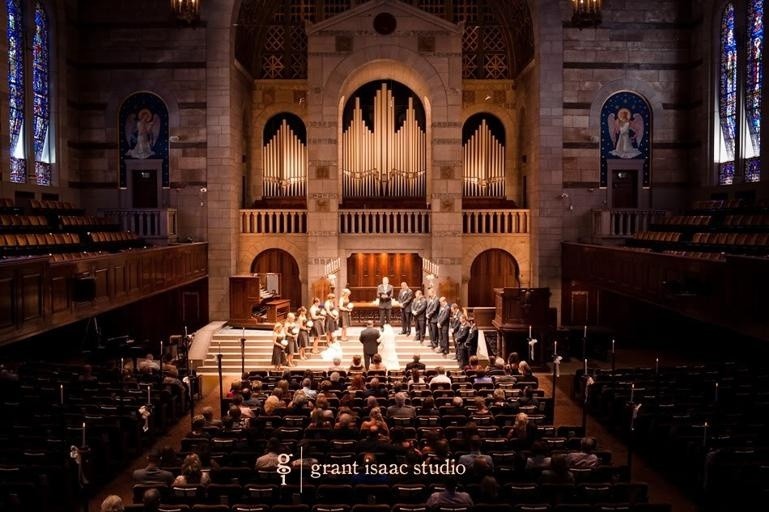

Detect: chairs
626;198;768;256
0;198;150;262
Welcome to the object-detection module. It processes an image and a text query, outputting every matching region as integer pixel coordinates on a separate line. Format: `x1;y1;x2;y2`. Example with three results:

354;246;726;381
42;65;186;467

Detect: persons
610;111;641;159
99;273;602;512
127;112;156;159
73;364;99;398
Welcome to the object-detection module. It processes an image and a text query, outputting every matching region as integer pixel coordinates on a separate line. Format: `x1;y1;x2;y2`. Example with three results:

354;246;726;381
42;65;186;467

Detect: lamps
169;0;208;31
570;1;603;31
